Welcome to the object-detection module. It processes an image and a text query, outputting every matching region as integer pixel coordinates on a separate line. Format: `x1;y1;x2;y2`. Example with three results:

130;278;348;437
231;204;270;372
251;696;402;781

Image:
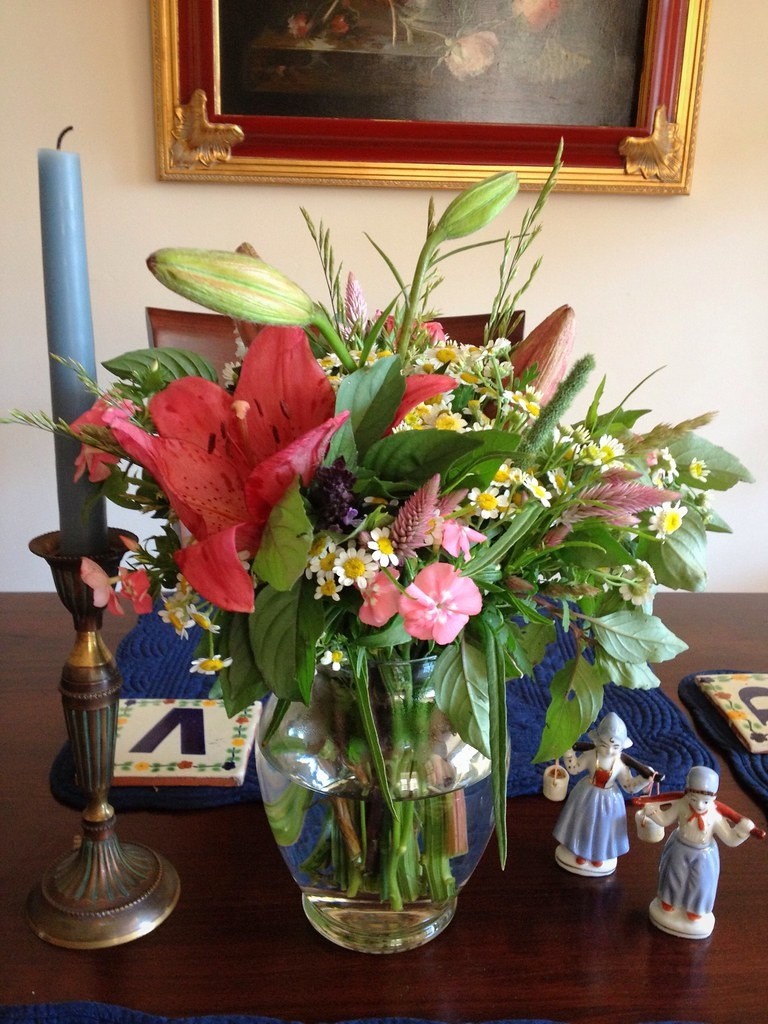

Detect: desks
1;590;768;1024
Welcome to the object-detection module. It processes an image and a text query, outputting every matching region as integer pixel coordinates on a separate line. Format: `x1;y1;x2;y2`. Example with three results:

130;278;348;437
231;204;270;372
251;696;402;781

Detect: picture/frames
149;1;711;196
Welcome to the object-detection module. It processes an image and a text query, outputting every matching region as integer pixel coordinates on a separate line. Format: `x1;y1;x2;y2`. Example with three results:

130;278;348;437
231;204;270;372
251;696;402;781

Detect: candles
38;125;107;555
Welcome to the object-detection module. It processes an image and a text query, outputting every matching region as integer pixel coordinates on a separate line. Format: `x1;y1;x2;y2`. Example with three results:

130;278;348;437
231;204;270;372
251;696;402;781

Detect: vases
254;649;509;954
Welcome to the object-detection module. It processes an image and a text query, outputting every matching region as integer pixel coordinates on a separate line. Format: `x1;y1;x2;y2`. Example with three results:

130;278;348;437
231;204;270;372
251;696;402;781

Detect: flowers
1;136;756;910
